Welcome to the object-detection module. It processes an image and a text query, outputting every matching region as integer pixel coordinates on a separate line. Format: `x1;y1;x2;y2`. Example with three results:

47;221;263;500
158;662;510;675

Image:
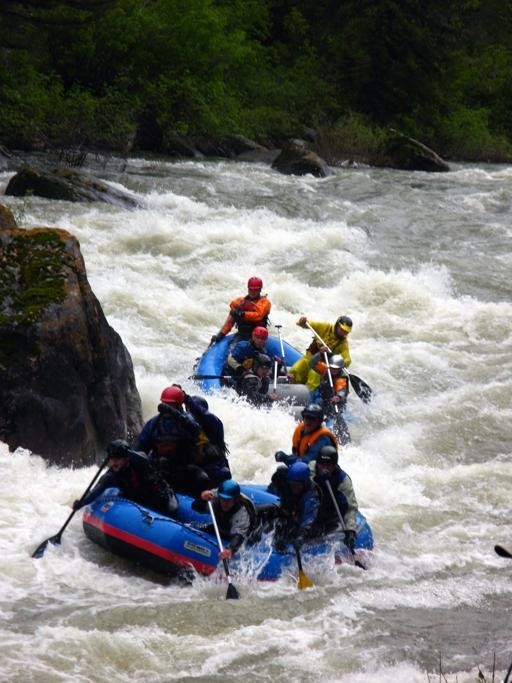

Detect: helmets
301;403;324;421
217;479;240;500
160;385;184;404
252;353;272;371
253;326;268;338
285;461;310;482
336;315;352;333
316;445;337;465
248;276;263;290
106;439;130;458
189;396;209;410
329;354;345;369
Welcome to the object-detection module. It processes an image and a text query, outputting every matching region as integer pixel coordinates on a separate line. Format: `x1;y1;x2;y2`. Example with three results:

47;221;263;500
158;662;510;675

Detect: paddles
323;351;351;446
206;498;241;598
324;479;366;571
294;541;313;588
305;321;372;405
187;374;294;381
32;456;109;558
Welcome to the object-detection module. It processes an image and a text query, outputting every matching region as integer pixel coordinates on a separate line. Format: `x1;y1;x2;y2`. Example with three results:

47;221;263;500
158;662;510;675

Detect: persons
192;479;252;561
70;384;230;503
237;349;275;408
216;276;268;336
287;314;352;390
272;402;338;467
272;459;316;550
306;443;357;550
305;346;350;407
222;329;267;383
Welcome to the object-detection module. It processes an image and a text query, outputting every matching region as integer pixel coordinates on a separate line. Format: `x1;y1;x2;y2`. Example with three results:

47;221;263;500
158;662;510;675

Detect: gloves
292;535;304;552
158;402;171;416
112;439;128;458
344;530;356;549
211;333;223;344
275;451;286;463
71;499;82;511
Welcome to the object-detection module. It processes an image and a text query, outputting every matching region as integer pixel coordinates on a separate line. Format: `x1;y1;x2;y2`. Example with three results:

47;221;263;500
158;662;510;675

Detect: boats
195;330;343;433
82;484;373;582
